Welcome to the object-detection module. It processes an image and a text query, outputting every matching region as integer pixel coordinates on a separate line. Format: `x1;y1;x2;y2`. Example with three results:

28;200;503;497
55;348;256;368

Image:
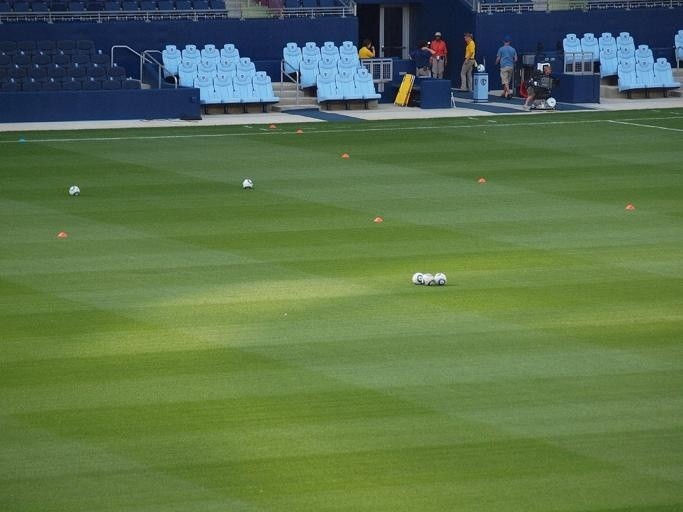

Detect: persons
429;32;448;79
459;33;476;92
522;66;556;111
409;40;436;77
358;39;376;58
494;35;518;100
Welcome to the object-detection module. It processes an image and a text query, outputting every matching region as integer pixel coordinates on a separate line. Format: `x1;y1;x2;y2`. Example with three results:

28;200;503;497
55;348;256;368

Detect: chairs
468;0;683;110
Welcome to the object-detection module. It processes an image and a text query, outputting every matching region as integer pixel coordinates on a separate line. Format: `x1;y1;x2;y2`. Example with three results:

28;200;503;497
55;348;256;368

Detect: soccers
476;64;485;73
412;272;446;287
69;187;78;195
242;178;255;187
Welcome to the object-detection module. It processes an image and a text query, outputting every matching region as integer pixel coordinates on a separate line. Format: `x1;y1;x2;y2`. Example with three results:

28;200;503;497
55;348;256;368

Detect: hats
503;36;512;42
435;32;441;37
